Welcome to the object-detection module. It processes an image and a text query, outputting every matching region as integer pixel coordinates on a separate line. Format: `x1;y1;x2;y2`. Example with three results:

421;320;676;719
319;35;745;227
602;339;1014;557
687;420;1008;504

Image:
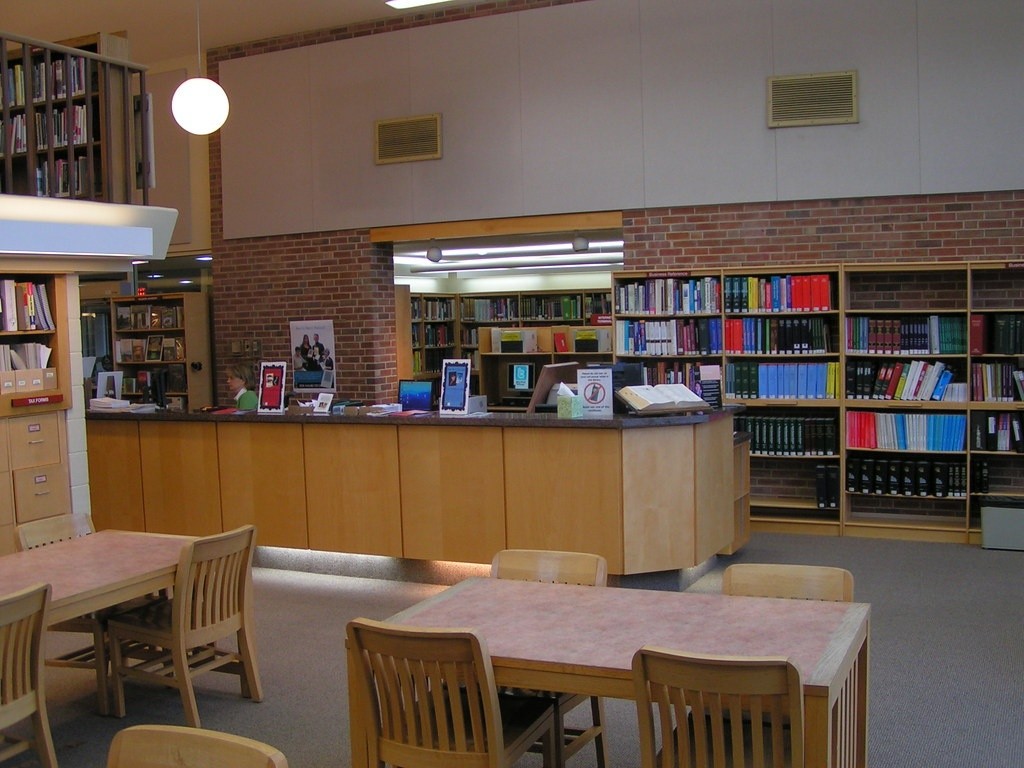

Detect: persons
294;334;333;371
225;364;259;410
449;372;456;386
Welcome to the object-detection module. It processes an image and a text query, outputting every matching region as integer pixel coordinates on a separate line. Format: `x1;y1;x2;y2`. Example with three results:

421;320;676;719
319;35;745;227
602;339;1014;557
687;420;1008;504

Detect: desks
717;431;754;555
0;530;207;644
377;576;871;768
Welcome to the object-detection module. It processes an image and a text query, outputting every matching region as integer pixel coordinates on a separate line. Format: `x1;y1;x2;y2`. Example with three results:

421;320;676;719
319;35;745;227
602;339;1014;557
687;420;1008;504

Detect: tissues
557;381;584;419
288;399;315;415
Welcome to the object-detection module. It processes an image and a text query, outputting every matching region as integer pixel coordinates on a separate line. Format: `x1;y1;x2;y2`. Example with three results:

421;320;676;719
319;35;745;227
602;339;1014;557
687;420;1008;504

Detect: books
0;56;88;200
846;313;1024;500
0;279;55;373
461;272;840;508
89;396;130;411
409;297;455;404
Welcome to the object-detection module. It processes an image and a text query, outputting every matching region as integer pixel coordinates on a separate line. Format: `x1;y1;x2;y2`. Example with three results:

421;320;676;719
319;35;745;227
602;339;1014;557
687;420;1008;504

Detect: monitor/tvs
142;368;168;406
586;361;645;415
397;379;435;412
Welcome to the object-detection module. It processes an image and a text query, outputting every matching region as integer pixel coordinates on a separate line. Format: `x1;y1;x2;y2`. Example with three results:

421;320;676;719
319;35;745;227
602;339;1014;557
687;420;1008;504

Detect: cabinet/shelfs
0;253;72;552
106;293;213;411
394;285;613;416
610;260;1024;548
0;30;132;206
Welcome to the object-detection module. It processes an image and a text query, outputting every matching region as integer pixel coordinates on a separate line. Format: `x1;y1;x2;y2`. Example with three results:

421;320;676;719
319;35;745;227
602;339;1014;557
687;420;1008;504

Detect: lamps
171;1;228;137
572;230;590;253
427;239;441;262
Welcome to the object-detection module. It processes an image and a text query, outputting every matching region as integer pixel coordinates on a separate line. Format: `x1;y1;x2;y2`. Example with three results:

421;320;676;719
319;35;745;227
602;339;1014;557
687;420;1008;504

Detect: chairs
487;549;607;767
0;584;61;768
14;512;168;717
108;725;286;768
107;524;257;729
344;616;557;768
631;647;806;767
718;564;856;611
